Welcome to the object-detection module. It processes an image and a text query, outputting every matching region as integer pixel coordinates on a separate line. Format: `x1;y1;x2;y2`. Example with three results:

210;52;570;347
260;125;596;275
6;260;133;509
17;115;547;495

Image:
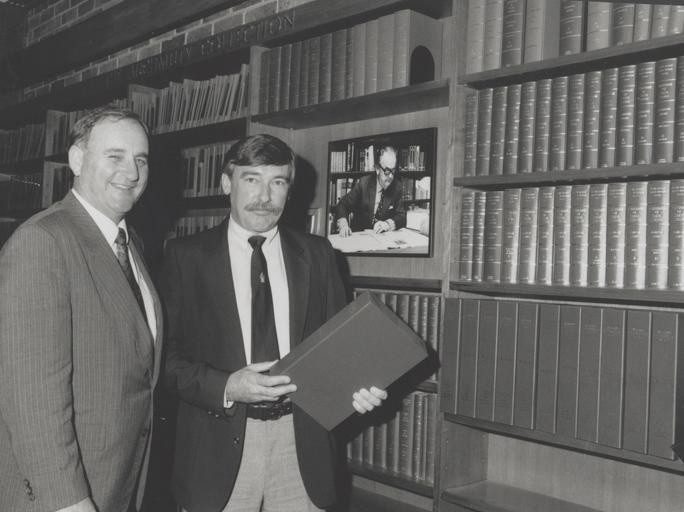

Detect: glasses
379;162;397;176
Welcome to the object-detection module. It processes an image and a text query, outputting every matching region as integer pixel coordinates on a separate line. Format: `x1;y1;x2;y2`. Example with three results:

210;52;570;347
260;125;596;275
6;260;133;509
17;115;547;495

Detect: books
345;287;441;489
458;179;681;293
260;9;443;116
330;145;430;235
1;83;136;244
124;44;266;245
464;4;684;176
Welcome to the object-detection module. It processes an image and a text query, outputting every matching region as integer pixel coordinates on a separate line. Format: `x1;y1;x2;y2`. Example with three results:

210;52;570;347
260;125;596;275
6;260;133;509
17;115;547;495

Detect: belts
245;404;291;419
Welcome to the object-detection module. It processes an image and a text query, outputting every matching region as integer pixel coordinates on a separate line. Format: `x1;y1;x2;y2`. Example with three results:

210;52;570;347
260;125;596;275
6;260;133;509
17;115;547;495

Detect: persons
158;133;387;511
0;105;165;511
331;143;406;239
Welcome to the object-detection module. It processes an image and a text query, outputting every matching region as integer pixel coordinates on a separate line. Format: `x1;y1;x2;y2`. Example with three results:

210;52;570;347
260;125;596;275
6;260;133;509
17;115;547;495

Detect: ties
116;231;148;319
247;236;279;363
375;189;385;224
